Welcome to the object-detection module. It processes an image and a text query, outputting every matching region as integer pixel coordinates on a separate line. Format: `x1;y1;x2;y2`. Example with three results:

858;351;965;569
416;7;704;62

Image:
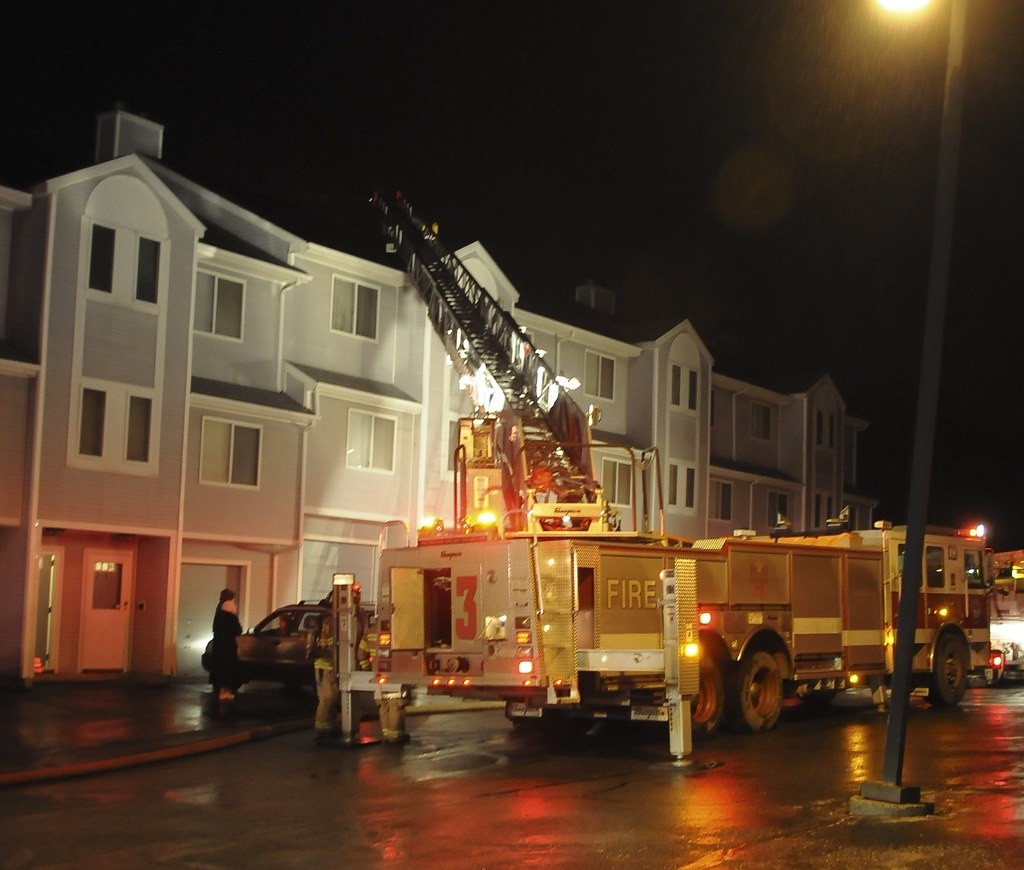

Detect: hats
220;588;235;601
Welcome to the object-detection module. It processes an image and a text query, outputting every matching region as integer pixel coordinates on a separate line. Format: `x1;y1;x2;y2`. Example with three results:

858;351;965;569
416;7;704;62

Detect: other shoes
220;692;235;699
315;721;332;729
386;733;410;743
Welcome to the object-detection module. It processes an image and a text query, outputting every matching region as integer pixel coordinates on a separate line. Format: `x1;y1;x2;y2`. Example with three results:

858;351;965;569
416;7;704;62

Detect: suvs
201;599;377;694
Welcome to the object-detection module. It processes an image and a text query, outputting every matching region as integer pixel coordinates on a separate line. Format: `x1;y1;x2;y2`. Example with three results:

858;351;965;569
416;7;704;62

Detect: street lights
858;0;970;816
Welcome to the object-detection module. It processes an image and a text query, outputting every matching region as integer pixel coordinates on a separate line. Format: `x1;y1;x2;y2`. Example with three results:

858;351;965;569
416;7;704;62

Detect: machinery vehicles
336;182;994;750
995;551;1024;682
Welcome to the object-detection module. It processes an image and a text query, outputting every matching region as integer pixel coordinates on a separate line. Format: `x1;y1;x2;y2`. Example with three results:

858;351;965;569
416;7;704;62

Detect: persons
208;590;243;700
356;610;412;745
306;593;343;740
